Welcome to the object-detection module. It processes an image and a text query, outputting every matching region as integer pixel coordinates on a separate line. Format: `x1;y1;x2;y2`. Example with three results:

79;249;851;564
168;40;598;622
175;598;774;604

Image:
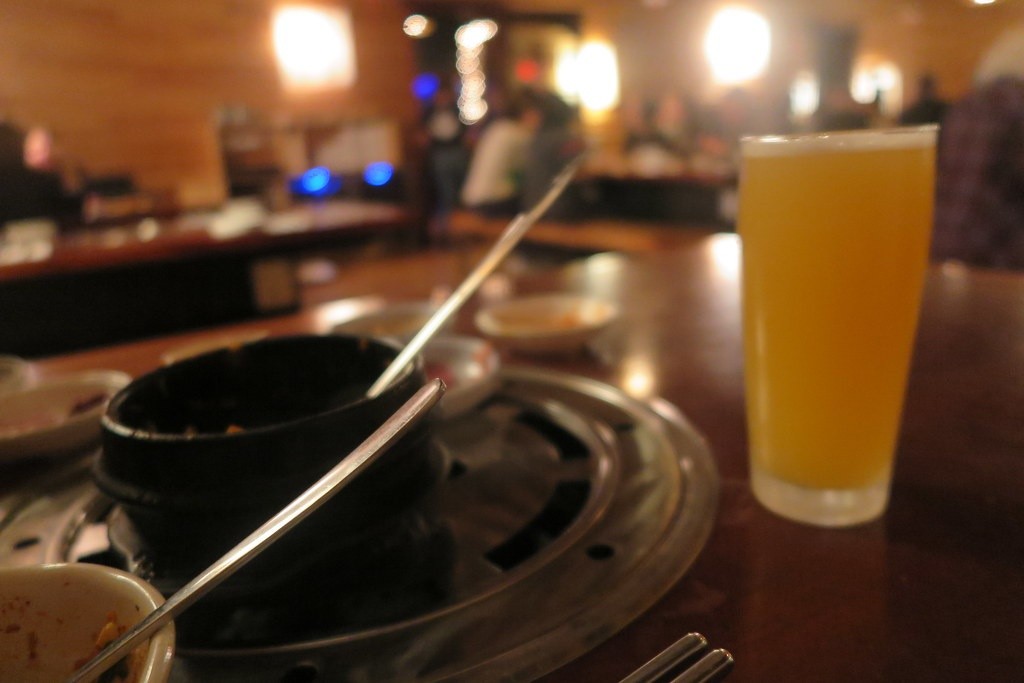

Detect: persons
420;79;697;220
0;120;98;231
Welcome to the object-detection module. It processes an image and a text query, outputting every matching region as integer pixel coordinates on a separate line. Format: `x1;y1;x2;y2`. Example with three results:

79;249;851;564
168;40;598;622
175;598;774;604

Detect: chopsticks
620;632;733;683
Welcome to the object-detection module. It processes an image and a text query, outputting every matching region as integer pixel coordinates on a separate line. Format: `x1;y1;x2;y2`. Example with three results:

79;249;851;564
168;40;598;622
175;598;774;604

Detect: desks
0;204;1024;683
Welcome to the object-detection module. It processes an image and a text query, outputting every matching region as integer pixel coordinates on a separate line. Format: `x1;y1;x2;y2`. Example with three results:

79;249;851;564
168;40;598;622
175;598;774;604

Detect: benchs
432;211;706;253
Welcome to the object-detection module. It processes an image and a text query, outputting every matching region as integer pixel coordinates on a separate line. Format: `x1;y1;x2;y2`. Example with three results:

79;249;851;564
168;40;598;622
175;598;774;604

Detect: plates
387;337;502;416
0;369;133;465
477;293;618;346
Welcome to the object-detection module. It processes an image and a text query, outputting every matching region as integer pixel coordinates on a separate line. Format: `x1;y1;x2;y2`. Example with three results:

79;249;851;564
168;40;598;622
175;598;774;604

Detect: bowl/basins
0;564;174;683
102;336;417;555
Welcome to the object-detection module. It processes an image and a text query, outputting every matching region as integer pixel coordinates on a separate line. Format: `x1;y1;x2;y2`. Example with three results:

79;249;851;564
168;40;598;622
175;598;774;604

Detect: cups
740;127;938;529
